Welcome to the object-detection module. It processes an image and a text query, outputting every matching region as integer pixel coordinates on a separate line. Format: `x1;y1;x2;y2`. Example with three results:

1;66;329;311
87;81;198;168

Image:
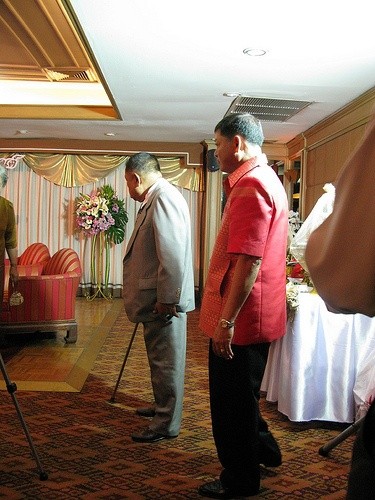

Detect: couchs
0;243;82;344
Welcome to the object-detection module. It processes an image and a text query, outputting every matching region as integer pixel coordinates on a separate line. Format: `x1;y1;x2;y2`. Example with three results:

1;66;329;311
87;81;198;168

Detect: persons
199;111;288;500
123;152;196;441
0;165;18;312
305;118;375;500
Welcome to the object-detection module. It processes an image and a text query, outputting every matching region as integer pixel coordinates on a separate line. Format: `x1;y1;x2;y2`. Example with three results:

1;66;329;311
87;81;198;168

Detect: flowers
286;209;303;324
68;184;128;247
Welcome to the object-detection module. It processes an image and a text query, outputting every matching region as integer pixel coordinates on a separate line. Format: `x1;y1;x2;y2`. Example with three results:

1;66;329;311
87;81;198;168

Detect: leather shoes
132;426;179;443
135;406;156;420
198;479;261;500
258;453;283;468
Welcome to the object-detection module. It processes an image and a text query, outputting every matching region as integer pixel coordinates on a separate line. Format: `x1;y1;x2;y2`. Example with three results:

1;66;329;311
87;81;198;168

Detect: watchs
218;318;234;330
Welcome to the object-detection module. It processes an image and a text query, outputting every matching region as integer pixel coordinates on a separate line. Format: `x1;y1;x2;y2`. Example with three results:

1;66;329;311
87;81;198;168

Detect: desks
260;277;375;424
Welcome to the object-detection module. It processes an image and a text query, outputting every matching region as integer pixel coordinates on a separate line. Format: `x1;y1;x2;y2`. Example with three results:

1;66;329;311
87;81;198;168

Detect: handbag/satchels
8;280;24;307
